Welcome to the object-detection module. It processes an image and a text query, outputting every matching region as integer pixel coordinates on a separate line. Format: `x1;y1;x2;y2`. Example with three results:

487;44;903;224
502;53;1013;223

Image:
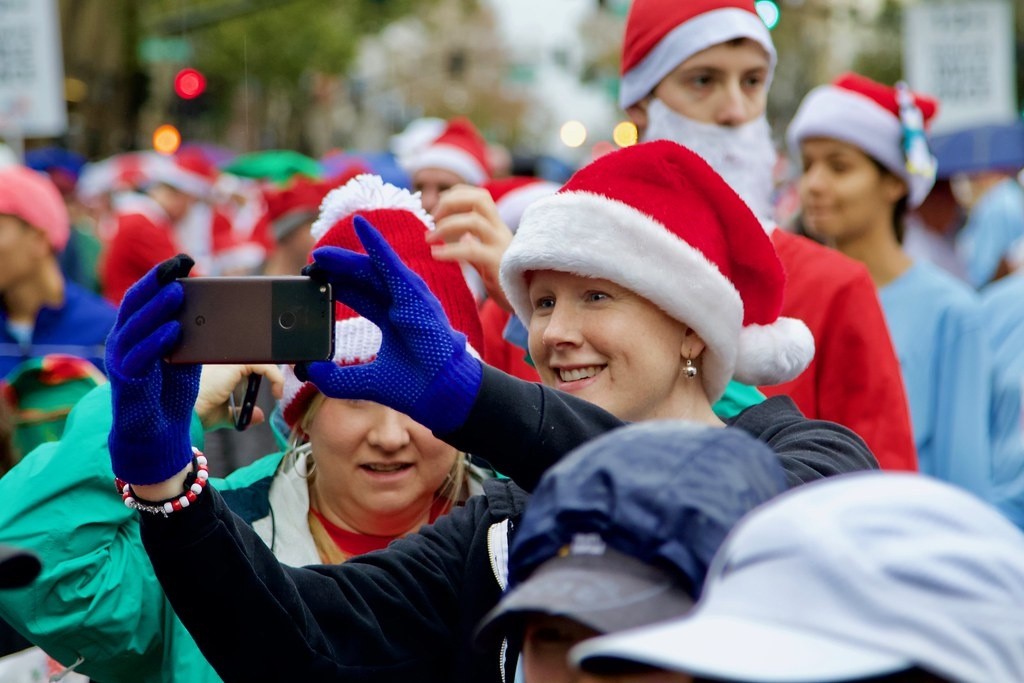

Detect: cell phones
163;274;337;365
231;372;264;432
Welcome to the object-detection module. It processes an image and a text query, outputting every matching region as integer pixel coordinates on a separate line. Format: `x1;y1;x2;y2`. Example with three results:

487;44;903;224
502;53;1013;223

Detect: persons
778;76;998;508
0;0;1024;683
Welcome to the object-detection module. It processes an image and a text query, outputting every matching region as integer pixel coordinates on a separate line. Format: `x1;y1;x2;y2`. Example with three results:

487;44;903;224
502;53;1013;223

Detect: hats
80;143;370;250
483;174;562;231
1;165;70;255
469;538;697;639
497;139;813;404
785;76;941;209
391;118;489;187
617;1;777;112
279;172;483;441
572;470;1024;683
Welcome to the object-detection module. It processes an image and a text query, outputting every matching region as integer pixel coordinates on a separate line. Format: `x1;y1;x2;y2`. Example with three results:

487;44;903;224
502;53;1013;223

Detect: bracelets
113;445;208;514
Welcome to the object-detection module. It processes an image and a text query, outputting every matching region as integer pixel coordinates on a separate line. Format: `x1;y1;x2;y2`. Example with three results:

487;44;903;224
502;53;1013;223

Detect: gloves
104;250;203;485
292;215;483;434
